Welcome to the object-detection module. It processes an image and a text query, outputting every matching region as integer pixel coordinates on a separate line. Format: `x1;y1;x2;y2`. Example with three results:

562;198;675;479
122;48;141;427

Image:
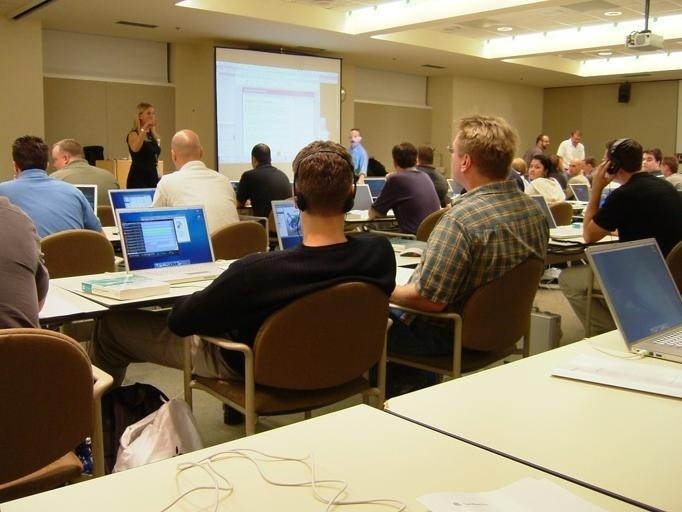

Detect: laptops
107;188;156;238
270;200;303;251
347;183;374;221
569;183;590;204
447;178;457;192
229;181;240;195
363;178;387;199
582;237;682;363
531;195;583;239
115;204;229;285
74;184;97;219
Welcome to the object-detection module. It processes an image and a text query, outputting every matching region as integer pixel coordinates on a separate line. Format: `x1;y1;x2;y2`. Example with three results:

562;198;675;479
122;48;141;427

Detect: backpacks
101;382;169;475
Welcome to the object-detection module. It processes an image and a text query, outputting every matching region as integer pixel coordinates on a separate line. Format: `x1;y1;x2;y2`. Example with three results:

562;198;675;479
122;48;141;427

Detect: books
74;272;170;301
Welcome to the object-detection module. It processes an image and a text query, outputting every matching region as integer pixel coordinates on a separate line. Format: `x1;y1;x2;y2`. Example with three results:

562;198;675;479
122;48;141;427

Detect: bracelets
141;129;150;133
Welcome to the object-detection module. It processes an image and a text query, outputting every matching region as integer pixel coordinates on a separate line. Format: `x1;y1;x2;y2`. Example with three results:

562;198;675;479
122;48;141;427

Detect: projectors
625;33;664;52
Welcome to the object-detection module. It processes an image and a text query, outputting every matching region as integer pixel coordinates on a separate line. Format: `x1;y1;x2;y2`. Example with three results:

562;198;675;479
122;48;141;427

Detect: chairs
0;328;115;503
551;201;573;226
266;209;278;242
96;205;115;226
83;145;104;166
584;239;682;338
181;282;394;437
386;256;545;379
40;229;116;280
210;220;268;260
371;208;451;242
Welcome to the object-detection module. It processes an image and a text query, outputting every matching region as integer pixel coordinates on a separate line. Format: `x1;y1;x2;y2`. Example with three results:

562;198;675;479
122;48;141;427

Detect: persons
583;136;681;260
237;143;292;217
371;112;549;378
1;136;108;240
641;147;661;176
346;128;368;184
151;129;239;235
660;157;681;190
125;104;160;189
523;134;550;165
564;160;591;200
583;156;596;175
510;158;528;183
546;152;567;190
370;143;441;234
88;141;396;388
556;129;586;170
417;146;449;201
0;195;50;329
49;139;119;206
525;155;566;204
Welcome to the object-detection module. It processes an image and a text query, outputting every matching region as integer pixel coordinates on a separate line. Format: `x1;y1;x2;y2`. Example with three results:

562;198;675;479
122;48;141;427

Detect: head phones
606;138;630;176
293;146;357;213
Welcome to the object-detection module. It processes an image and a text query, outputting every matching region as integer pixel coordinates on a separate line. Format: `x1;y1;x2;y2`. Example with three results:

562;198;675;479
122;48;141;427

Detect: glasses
447;146;458;153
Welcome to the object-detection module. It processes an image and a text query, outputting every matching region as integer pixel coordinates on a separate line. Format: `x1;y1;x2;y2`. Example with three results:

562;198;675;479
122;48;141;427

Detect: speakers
618;84;631;103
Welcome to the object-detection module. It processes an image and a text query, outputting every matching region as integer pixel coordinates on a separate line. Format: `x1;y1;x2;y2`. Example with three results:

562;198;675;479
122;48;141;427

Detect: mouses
399;248;423;258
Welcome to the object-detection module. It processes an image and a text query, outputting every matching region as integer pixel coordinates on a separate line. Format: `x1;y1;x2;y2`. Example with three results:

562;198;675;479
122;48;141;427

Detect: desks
36;282;111;364
1;402;652;512
101;225;121;241
48;251;423;343
344;217;399;237
383;328;681;512
528;224;620;351
567;200;589;219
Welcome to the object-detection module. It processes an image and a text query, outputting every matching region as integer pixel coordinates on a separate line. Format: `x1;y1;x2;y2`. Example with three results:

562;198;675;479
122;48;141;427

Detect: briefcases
528;306;561;355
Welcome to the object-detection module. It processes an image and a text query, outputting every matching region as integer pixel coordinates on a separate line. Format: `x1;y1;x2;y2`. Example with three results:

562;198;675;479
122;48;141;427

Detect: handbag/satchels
111;398;203;473
